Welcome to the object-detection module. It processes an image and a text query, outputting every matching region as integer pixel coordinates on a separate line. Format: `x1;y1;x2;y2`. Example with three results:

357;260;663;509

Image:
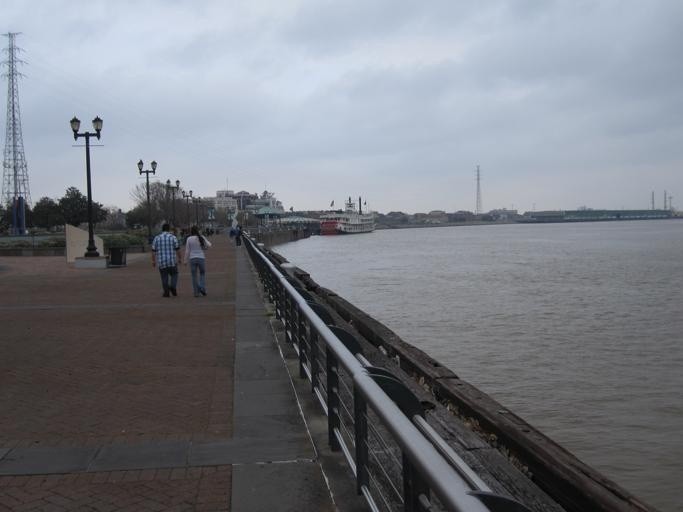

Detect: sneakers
163;292;170;297
200;288;207;296
195;293;201;297
170;287;177;296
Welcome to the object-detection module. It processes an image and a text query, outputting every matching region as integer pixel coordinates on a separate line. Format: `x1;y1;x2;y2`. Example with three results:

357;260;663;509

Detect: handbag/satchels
200;235;208;250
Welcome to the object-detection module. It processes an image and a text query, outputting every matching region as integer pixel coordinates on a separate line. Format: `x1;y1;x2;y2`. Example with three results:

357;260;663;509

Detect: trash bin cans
105;238;127;268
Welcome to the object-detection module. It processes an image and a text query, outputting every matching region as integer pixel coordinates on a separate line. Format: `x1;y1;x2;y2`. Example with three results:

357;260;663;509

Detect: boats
317;195;376;236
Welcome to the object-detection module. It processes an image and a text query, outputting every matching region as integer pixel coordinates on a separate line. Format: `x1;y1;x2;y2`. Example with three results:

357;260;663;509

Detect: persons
183;224;212;297
150;222;182;298
228;225;243;249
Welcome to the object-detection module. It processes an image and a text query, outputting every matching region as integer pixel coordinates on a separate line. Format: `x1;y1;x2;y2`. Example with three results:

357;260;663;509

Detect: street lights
69;115;103;258
165;179;179;237
136;160;157;242
181;189;213;234
